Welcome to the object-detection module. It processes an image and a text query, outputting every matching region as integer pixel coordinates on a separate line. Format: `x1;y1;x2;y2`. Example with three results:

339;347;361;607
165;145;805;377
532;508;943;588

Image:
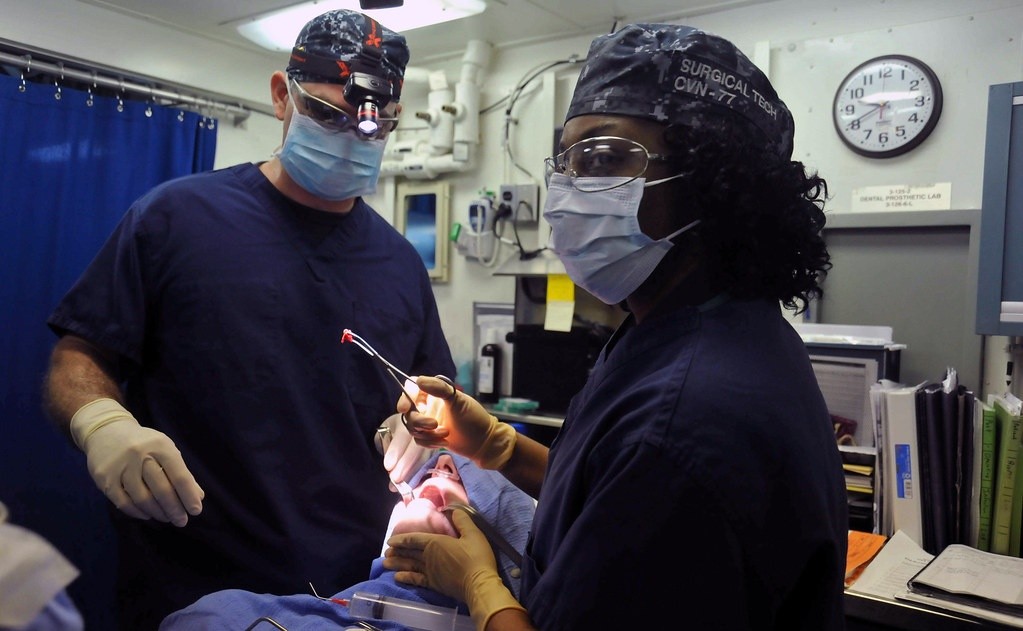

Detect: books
869;368;1023;631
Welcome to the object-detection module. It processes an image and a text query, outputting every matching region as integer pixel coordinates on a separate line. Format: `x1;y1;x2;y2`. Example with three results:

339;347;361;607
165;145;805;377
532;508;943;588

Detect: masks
542;173;702;306
274;82;390;202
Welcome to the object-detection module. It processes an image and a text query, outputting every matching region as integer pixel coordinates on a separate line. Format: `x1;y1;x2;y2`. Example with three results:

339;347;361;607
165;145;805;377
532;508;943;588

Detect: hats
287;8;409;103
562;23;795;175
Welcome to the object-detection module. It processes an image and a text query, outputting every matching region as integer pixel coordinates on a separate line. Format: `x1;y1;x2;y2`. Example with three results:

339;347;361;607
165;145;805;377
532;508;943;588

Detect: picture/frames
397;182;449;281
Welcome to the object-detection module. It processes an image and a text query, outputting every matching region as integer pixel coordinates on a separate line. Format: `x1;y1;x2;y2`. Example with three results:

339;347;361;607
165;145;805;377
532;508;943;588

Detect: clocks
832;54;944;159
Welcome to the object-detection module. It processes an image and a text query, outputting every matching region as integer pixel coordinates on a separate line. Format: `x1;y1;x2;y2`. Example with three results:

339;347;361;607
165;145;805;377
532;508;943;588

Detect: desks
480;403;566;428
843;589;1005;631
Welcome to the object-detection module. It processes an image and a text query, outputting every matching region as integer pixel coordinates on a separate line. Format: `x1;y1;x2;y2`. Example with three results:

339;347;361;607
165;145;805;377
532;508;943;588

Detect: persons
162;450;536;631
398;21;847;631
45;9;457;617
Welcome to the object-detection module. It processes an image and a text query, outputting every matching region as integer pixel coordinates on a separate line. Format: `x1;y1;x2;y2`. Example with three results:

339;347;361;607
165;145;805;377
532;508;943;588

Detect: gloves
383;509;528;631
70;398;205;528
375;412;433;492
397;375;517;470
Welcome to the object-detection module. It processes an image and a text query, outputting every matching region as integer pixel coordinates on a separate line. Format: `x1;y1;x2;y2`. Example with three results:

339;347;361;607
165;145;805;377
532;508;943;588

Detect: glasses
544;136;679;193
286;78;399;141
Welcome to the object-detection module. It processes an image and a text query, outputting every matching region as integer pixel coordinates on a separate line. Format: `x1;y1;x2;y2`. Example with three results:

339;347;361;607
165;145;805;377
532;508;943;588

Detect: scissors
348;331;457;433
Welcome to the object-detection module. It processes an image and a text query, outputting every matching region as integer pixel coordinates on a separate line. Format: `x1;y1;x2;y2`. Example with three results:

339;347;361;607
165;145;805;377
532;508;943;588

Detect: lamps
220;0;509;52
